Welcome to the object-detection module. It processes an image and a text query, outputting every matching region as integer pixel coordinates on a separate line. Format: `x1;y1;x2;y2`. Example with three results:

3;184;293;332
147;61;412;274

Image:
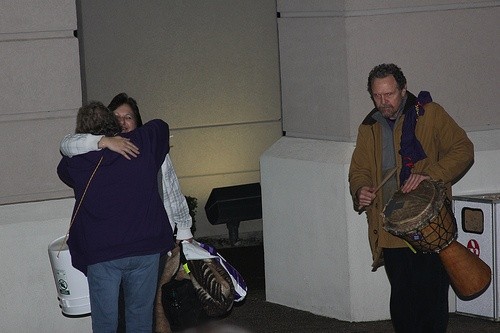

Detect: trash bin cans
450;192;500;322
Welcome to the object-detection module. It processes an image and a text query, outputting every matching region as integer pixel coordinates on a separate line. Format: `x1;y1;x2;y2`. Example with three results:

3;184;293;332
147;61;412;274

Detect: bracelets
110;137;113;150
103;137;106;148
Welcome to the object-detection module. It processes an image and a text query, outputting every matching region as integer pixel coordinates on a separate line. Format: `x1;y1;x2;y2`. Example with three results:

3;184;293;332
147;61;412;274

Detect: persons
56;100;170;333
348;63;475;333
60;92;194;333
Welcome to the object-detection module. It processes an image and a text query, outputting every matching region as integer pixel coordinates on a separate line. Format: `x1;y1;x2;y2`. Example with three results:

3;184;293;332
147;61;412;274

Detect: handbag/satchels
161;238;248;333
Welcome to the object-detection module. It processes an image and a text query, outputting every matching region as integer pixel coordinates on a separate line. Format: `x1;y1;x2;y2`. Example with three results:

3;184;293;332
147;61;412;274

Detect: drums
379;179;493;301
